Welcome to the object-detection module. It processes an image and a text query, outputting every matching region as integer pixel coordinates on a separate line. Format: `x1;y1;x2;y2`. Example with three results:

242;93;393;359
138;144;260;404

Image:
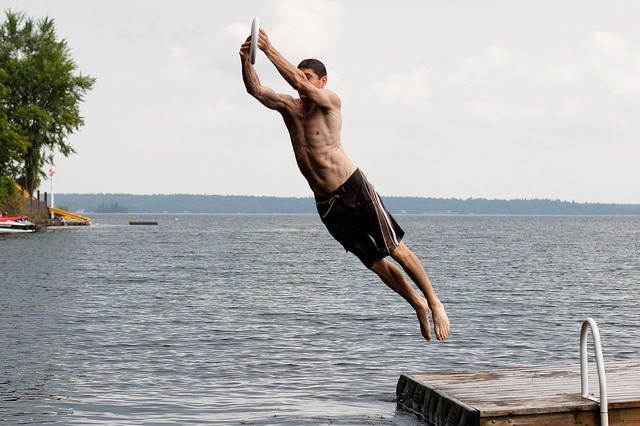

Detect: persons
239;28;450;342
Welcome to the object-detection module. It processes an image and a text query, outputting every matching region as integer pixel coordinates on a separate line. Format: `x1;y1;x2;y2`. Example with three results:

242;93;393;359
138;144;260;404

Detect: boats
7;216;36;232
0;212;27;233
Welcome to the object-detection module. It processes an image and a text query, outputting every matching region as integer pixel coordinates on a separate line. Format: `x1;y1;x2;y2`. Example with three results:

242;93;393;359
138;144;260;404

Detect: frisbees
250;17;260;65
